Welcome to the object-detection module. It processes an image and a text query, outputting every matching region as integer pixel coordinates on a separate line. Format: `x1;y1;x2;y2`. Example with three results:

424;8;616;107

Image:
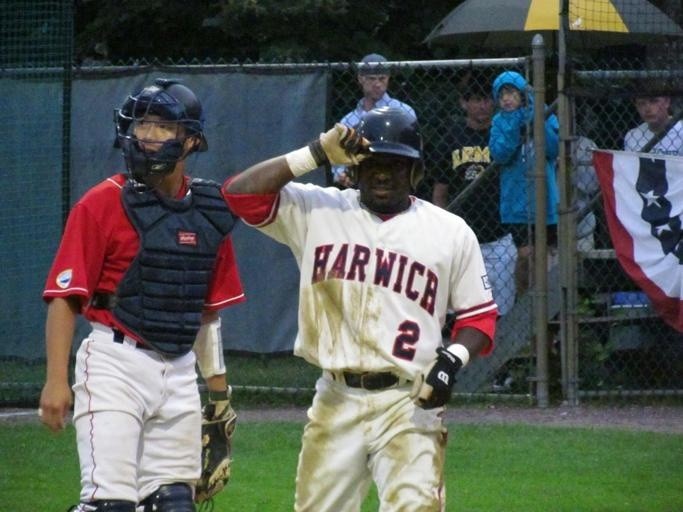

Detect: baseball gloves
193;404;237;503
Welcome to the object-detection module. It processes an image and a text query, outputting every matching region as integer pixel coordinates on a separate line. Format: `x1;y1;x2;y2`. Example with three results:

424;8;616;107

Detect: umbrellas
413;2;683;54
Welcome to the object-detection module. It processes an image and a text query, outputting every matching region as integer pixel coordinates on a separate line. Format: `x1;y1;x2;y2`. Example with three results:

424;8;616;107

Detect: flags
589;150;683;336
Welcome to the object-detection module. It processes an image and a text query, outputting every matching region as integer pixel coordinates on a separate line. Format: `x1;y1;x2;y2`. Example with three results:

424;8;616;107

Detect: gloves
409;347;462;408
308;123;370;166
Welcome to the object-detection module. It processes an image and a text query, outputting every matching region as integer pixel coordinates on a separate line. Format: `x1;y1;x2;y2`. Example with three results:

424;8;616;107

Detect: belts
331;370;398;390
113;329;151;349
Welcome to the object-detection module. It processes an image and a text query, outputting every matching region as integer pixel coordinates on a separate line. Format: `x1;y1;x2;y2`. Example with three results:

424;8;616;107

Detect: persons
624;83;682;157
429;77;499;243
327;51;425;190
571;134;598;251
31;78;250;512
487;68;560;248
217;102;500;512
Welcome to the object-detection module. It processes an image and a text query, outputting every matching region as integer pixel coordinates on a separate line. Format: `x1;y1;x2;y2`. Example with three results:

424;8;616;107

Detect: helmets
113;78;208;177
356;106;424;158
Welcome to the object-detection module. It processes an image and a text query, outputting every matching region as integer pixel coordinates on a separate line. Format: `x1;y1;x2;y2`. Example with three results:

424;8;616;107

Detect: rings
37;407;43;417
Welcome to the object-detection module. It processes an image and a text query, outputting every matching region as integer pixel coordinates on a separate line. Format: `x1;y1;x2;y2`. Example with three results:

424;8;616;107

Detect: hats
360;54;391;74
